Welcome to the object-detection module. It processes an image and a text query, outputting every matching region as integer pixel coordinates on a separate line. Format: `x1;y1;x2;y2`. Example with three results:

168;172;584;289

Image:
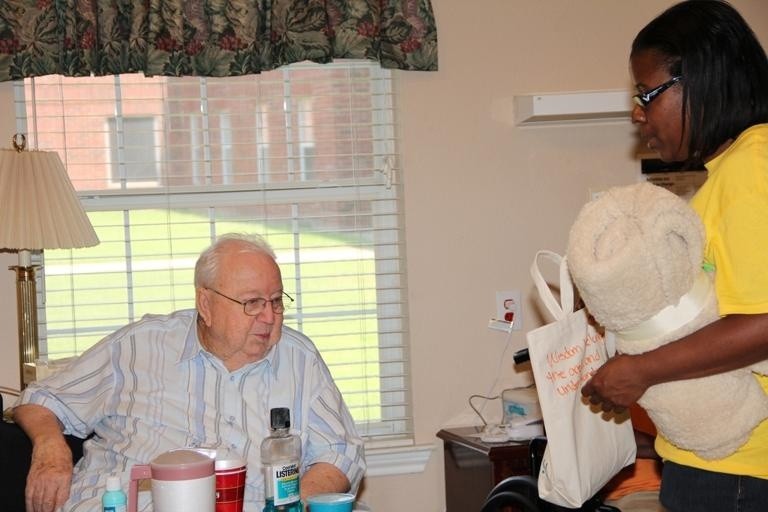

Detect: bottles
101;475;127;512
129;449;215;511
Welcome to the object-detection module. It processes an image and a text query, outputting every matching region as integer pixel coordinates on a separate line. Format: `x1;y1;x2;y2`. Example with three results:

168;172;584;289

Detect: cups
214;460;248;511
305;492;356;512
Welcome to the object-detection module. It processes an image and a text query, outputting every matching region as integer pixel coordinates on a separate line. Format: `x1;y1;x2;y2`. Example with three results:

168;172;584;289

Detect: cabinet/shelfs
435;424;546;512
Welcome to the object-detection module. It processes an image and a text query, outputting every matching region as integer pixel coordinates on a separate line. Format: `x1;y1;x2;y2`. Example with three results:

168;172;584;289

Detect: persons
10;230;366;512
578;1;768;511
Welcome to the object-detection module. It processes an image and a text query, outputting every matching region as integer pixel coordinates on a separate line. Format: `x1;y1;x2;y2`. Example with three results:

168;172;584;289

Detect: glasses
630;76;683;109
205;286;295;320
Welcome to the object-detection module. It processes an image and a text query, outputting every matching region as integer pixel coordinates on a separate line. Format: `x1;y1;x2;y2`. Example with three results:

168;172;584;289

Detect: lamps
515;89;640;128
0;133;101;395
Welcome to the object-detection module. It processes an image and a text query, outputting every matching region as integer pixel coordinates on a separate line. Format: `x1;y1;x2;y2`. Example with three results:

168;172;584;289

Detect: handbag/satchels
523;243;639;507
